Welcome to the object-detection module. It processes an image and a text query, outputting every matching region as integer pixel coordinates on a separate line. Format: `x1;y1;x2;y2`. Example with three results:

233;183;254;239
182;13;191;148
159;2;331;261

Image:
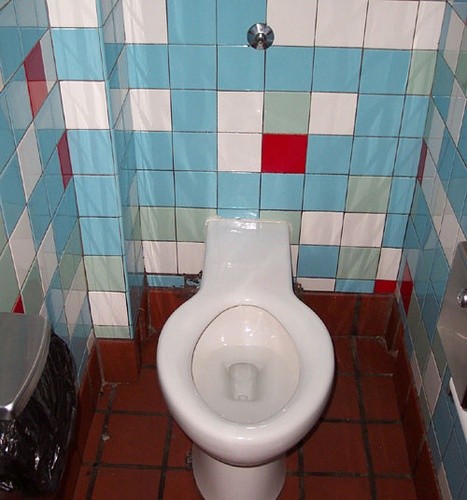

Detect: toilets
155;220;336;500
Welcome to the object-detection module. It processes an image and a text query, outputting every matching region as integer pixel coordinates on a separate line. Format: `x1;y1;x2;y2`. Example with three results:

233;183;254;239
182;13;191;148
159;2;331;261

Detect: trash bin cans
0;311;76;500
